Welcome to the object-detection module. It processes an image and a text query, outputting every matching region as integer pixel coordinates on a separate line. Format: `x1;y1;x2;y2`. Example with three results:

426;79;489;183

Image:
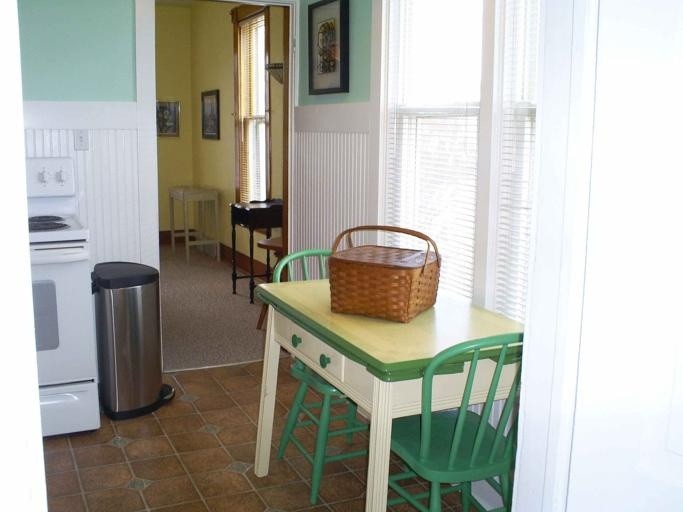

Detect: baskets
328;225;442;323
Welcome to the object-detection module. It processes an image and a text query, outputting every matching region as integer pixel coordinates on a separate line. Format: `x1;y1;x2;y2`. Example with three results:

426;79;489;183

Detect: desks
169;186;221;262
229;199;282;305
254;280;520;512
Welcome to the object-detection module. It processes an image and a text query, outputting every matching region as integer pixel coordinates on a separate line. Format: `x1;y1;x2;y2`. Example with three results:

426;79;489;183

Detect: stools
257;234;283;329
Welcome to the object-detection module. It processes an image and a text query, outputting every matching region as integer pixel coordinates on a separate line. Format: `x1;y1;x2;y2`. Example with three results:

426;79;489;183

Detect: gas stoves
30;213;88;242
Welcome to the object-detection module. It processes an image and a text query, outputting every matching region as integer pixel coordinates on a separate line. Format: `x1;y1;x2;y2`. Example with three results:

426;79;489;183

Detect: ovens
29;246;100;388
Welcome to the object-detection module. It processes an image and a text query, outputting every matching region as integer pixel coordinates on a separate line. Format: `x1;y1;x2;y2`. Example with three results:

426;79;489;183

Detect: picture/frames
308;0;350;96
156;100;180;137
200;88;220;141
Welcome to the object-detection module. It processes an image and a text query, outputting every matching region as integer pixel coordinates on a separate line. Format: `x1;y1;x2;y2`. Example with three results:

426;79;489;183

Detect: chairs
389;329;524;512
274;248;371;505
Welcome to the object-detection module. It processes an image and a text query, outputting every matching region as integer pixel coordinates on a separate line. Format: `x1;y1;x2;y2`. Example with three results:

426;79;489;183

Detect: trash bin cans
91;261;175;420
